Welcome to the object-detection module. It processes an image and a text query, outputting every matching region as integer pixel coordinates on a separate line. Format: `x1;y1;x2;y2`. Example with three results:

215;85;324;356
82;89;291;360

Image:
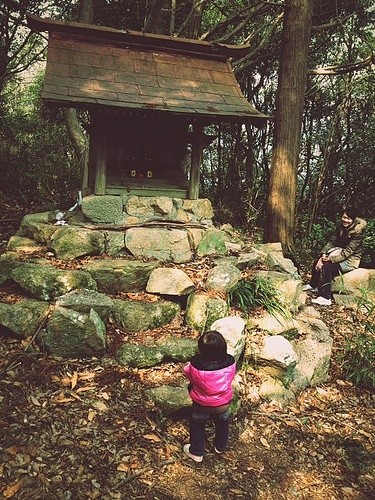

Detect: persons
181;330;237;463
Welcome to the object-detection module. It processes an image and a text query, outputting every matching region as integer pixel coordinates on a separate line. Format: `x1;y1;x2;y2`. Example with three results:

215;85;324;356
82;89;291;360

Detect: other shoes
302;284;318;293
183;444;203;462
214;447;224;454
311;296;331;305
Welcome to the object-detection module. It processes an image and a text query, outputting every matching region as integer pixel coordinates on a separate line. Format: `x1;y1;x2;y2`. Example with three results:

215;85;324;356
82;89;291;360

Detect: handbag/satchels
326;246;345;257
